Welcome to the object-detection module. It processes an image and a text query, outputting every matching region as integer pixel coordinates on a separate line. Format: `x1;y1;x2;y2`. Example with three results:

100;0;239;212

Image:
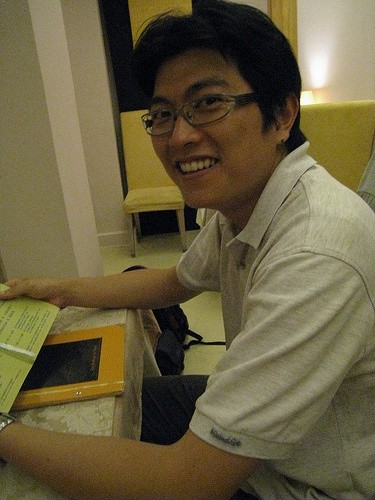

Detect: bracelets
0;411;16;469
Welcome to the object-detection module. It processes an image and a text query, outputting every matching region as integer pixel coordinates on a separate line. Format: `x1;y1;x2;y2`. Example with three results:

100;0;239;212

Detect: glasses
142;87;268;135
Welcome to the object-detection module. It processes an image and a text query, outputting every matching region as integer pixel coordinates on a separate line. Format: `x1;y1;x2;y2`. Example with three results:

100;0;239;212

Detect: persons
0;2;373;500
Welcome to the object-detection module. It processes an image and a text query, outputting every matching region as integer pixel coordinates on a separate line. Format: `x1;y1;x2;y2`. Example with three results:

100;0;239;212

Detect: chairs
120;109;188;255
302;99;375;195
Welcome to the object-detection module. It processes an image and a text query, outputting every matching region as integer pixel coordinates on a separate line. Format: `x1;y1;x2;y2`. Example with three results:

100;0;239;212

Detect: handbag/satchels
155;327;183;374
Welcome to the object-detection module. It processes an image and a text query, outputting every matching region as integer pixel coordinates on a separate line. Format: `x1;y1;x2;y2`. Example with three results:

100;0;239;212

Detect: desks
0;304;165;500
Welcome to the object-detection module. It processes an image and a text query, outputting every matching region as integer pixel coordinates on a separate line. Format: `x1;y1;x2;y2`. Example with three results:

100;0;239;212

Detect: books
0;282;59;415
9;322;128;411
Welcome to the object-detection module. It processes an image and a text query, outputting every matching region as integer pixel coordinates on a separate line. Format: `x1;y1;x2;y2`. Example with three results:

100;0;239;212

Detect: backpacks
123;264;205;341
